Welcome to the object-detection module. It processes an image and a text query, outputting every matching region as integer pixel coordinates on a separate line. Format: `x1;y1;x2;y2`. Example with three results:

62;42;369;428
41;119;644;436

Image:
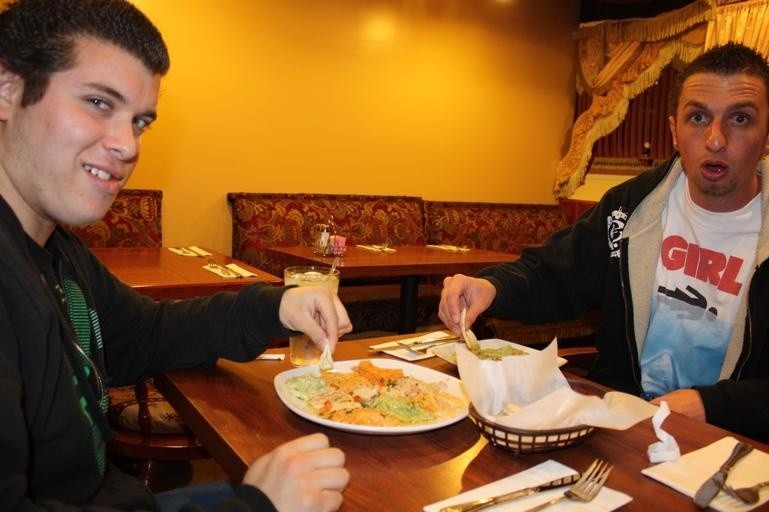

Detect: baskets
470;379;606;454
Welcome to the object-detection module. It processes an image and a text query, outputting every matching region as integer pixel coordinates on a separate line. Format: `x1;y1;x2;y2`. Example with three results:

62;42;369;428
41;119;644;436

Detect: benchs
227;191;605;345
57;188;165;245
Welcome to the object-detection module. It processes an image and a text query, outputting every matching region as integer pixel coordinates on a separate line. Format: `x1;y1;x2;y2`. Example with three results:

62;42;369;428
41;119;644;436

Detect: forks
207;257;232;276
395;342;459;359
524;456;616;511
715;474;768;506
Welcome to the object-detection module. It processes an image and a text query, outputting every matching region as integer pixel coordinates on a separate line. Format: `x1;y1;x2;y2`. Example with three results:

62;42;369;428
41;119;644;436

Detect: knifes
214;259;241;280
366;333;454;353
441;468;581;512
691;439;752;505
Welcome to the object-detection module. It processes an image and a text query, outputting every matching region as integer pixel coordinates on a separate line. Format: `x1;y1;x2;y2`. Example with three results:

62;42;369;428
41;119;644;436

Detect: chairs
107;378;215;494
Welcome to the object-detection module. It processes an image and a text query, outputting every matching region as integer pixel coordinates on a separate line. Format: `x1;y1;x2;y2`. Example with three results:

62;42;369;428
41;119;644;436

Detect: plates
434;339;571;368
639;433;769;510
272;357;471;438
420;457;633;511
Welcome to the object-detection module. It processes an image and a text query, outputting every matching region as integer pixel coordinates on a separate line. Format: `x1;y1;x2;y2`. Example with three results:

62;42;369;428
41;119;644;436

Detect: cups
285;262;344;371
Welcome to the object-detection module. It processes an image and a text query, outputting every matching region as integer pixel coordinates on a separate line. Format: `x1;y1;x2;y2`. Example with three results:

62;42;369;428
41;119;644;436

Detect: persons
437;38;769;437
0;0;355;511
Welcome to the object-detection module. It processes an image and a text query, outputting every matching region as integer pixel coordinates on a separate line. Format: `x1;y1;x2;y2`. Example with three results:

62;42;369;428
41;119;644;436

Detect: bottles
314;222;330;251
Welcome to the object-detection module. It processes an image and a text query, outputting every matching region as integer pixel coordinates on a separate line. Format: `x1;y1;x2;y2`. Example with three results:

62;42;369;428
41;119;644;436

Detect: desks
262;241;520;335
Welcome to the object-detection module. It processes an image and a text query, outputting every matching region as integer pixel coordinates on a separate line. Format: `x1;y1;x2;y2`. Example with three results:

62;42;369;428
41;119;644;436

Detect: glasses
40;269;104;404
